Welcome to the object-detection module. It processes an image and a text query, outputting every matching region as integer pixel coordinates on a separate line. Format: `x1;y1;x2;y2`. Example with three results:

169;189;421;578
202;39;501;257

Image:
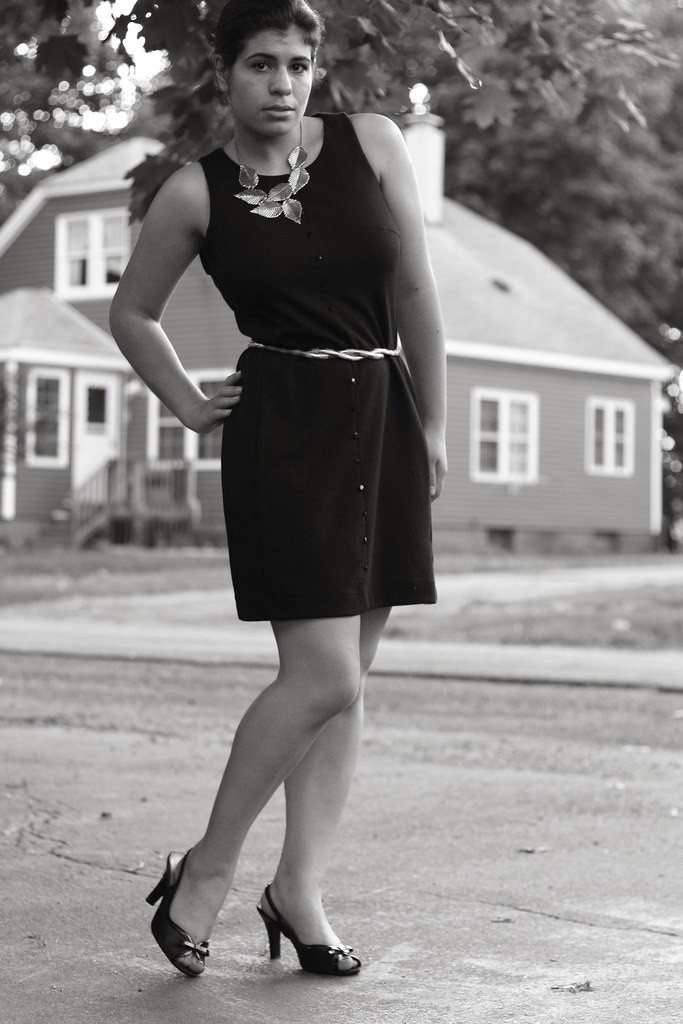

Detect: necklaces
230;120;309;224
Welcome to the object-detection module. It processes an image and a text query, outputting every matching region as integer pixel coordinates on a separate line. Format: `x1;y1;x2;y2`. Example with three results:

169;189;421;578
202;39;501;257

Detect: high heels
258;881;362;976
145;851;212;979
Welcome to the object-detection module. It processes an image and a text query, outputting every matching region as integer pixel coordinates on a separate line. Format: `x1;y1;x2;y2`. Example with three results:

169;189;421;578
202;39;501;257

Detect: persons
108;0;446;977
